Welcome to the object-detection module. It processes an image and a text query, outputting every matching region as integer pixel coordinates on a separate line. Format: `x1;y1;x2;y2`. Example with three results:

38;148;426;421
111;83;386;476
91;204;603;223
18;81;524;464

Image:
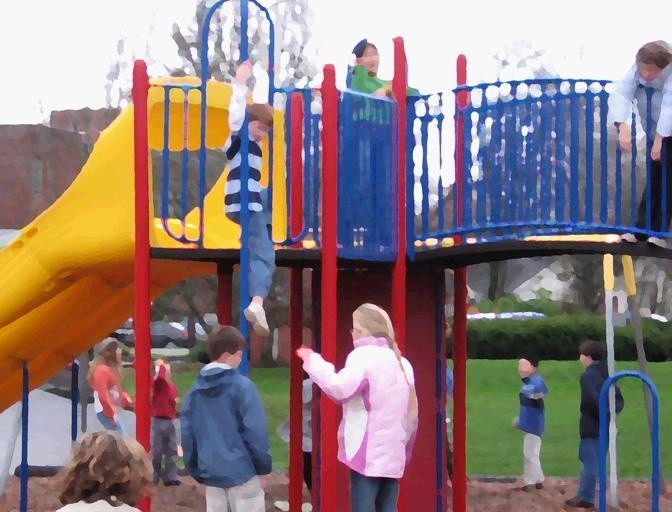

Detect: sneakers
243;301;270;338
